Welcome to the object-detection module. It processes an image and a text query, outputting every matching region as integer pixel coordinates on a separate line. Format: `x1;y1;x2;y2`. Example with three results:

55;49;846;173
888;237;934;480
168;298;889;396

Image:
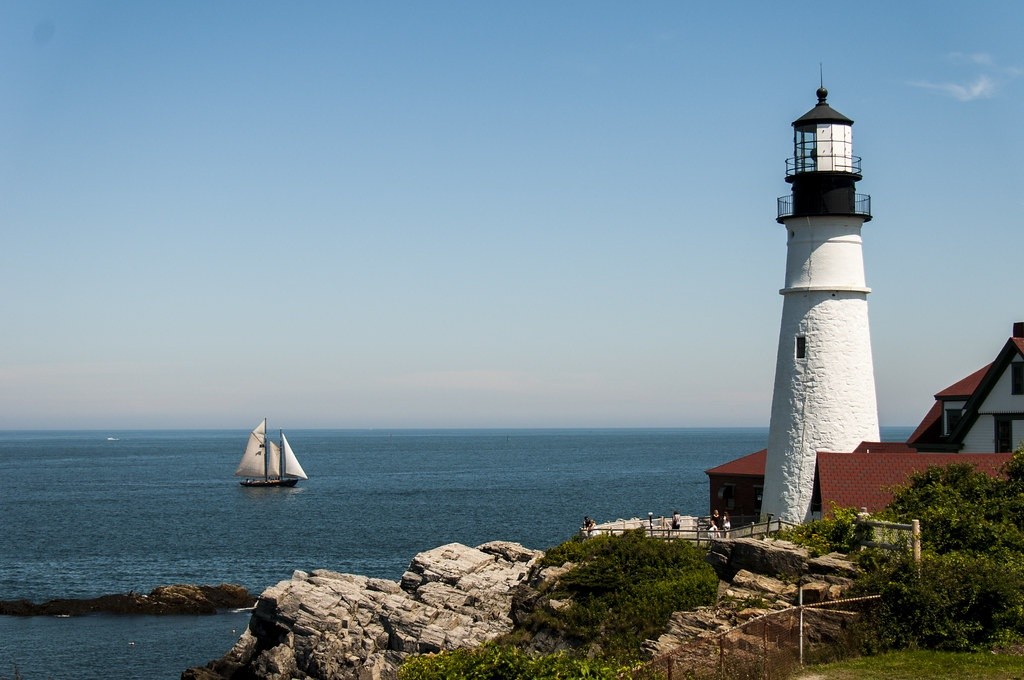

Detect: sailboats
234;418;311;487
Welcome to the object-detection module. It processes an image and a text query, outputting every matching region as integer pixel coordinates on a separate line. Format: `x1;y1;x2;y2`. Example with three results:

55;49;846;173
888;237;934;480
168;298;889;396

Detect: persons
673;511;681;529
583;516;592;535
246;479;249;483
711;510;721;530
706;520;721;538
587;519;598;536
721;512;731;538
